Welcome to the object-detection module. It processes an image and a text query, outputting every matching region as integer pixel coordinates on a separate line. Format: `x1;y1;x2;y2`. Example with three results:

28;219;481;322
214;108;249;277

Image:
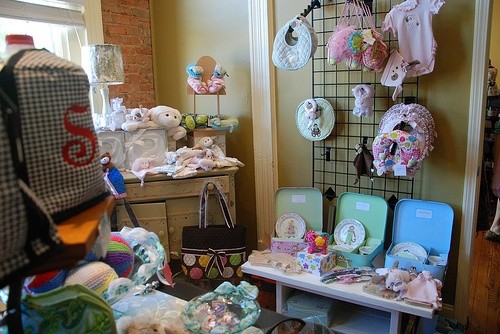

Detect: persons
484;58;500;121
483;116;500;242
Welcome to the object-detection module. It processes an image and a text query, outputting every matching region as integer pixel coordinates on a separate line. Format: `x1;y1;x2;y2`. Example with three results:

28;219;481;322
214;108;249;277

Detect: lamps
81;43;125;114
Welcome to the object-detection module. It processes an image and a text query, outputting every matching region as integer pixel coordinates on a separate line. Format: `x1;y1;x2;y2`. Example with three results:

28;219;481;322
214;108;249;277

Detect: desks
241;249;434;334
112;264;346;334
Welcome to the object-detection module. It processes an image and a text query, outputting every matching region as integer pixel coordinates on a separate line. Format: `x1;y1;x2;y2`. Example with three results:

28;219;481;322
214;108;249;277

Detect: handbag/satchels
0;47;112;288
1;284;117;334
181;178;247;283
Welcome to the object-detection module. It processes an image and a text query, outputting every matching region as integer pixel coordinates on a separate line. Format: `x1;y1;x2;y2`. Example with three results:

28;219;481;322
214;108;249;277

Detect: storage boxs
385;198;455;281
329;191;389;268
297;246;336;276
176;128;226;157
270;186;324;255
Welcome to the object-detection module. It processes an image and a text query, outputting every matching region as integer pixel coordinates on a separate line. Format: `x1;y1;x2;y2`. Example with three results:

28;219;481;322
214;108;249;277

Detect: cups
429;256;443;264
360;246;371;255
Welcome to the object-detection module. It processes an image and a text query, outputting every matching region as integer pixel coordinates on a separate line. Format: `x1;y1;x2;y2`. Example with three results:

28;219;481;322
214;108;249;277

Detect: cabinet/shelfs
116;172;236;263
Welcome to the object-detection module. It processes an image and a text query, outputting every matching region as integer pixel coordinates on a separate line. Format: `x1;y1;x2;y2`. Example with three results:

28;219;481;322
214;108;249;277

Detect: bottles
4;34;35;65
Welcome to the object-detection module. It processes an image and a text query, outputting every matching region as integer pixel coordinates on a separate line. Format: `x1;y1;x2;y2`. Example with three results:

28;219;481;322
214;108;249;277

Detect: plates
275;213;307;239
334;219;366;248
180;291;261;334
390;242;427;264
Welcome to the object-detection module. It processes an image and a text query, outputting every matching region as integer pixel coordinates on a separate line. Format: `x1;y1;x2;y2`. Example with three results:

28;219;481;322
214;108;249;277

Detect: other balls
85;232;134;278
22;269;68;295
65;261;119;296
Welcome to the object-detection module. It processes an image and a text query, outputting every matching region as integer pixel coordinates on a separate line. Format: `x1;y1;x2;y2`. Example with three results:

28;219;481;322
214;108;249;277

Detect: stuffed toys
185;63;229;93
329;26;388;73
101;150;114;173
173;136;225;171
122;106;186;140
353;85;374;118
181;113;207;130
